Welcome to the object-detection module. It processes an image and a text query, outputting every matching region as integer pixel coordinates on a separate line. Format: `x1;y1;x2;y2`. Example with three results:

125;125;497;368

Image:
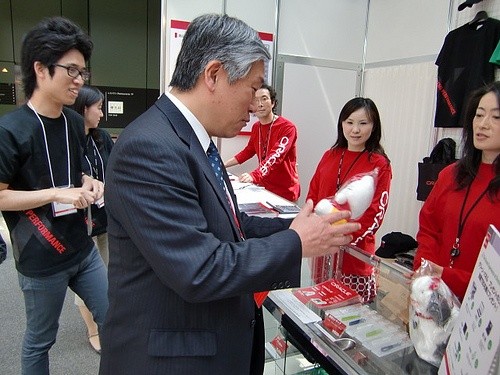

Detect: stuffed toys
408;276;462;367
313;176;375;237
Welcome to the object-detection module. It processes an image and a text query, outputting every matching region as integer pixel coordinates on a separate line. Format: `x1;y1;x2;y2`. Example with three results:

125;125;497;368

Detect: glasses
47;62;91;81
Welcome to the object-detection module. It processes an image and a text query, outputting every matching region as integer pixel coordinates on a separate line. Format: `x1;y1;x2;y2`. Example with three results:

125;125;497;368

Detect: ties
205;140;233;212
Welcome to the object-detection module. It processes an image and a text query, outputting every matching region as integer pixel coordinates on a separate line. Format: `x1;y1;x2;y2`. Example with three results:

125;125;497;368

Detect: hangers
468;11;495;25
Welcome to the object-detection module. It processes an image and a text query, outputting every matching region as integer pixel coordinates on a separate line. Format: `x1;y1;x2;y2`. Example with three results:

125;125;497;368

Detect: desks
226;170;300;218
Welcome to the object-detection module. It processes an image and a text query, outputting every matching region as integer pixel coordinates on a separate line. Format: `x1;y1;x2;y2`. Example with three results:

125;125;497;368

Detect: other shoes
86;329;101;354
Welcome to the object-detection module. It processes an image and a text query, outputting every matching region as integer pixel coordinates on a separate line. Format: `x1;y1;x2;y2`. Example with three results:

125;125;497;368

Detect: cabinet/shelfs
257;245;458;375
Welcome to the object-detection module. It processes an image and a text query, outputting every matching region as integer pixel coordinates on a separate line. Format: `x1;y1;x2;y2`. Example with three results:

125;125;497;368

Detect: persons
412;79;500;299
69;85;114;354
99;13;361;375
222;84;301;204
0;17;108;375
304;97;392;305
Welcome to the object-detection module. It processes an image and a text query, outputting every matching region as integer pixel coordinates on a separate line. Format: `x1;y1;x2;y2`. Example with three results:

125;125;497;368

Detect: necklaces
260;116;276;159
337;148;365;191
448;181;489;257
